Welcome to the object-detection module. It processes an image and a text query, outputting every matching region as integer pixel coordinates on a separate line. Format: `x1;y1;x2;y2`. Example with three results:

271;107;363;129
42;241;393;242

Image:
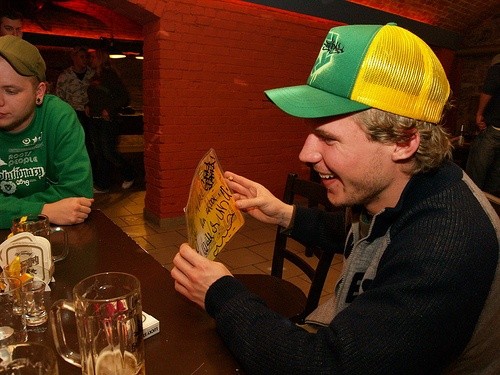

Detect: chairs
231;172;346;326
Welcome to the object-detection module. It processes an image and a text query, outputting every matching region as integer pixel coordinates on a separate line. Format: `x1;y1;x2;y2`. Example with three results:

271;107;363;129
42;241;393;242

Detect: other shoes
93;179;134;193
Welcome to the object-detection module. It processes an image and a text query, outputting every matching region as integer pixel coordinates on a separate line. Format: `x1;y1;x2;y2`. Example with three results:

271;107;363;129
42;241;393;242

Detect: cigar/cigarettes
227;175;233;180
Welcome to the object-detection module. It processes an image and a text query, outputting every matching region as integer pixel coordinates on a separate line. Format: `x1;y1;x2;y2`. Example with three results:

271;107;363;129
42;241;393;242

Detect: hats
263;22;451;125
0;34;47;81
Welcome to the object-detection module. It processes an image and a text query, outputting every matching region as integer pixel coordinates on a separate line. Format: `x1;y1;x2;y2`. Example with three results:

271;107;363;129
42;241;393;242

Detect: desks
0;209;249;375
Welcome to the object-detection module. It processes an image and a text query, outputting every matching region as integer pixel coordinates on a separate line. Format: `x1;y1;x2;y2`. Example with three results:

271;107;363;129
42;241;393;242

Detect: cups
14;215;70;279
21;281;49;327
0;277;31;349
49;271;146;375
0;342;61;375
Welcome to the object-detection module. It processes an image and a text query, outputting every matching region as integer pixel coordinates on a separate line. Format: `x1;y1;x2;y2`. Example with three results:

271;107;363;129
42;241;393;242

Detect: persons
0;33;95;227
473;46;499;193
170;22;500;375
56;44;99;195
88;44;133;194
